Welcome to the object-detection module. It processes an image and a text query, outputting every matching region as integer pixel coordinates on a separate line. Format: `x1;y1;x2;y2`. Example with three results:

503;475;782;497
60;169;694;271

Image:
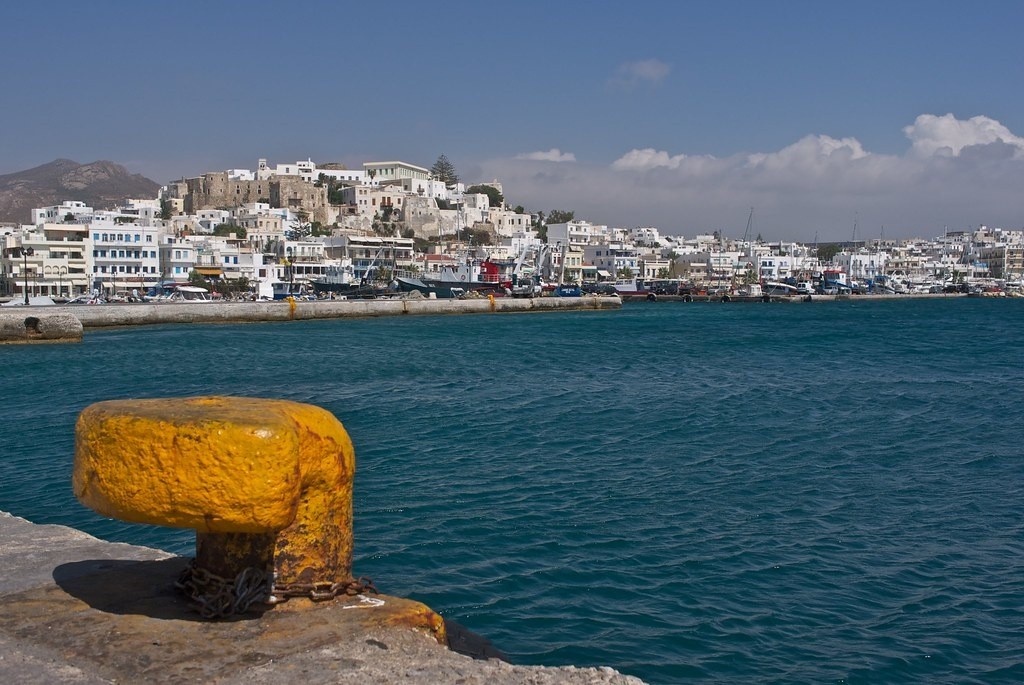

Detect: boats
0;206;1024;305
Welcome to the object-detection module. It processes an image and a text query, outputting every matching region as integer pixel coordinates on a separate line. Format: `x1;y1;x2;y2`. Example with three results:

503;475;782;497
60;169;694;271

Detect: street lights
138;273;144;295
57;271;64;298
111;272;118;295
85;273;93;294
19;245;34;305
